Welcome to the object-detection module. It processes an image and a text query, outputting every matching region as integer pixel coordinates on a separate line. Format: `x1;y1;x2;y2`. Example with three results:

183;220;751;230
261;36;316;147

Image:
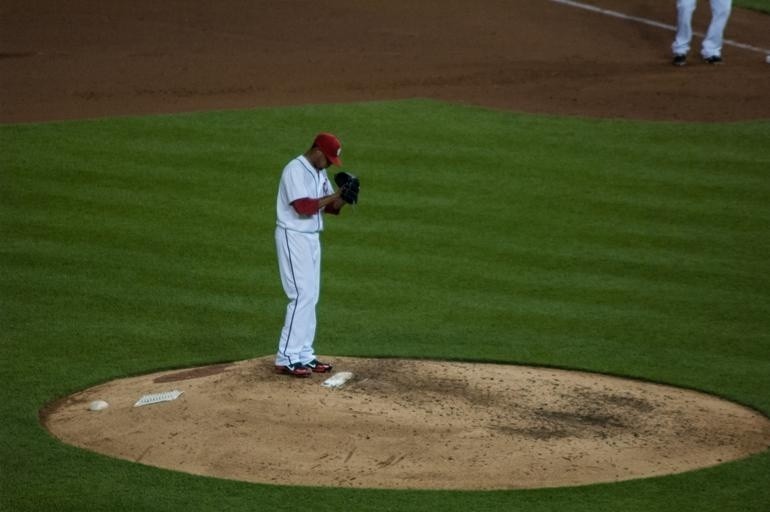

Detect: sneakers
274;361;311;377
702;53;723;65
302;359;332;373
671;54;687;67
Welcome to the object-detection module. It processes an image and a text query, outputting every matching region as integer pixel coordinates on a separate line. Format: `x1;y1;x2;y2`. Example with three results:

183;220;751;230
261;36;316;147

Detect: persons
671;0;733;66
274;132;359;375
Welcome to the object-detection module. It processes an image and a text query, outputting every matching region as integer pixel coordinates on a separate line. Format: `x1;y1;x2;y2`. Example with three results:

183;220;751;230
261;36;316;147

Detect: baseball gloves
334;172;360;203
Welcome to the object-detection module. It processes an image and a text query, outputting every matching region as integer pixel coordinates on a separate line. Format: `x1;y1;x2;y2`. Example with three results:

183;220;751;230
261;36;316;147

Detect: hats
313;131;344;168
